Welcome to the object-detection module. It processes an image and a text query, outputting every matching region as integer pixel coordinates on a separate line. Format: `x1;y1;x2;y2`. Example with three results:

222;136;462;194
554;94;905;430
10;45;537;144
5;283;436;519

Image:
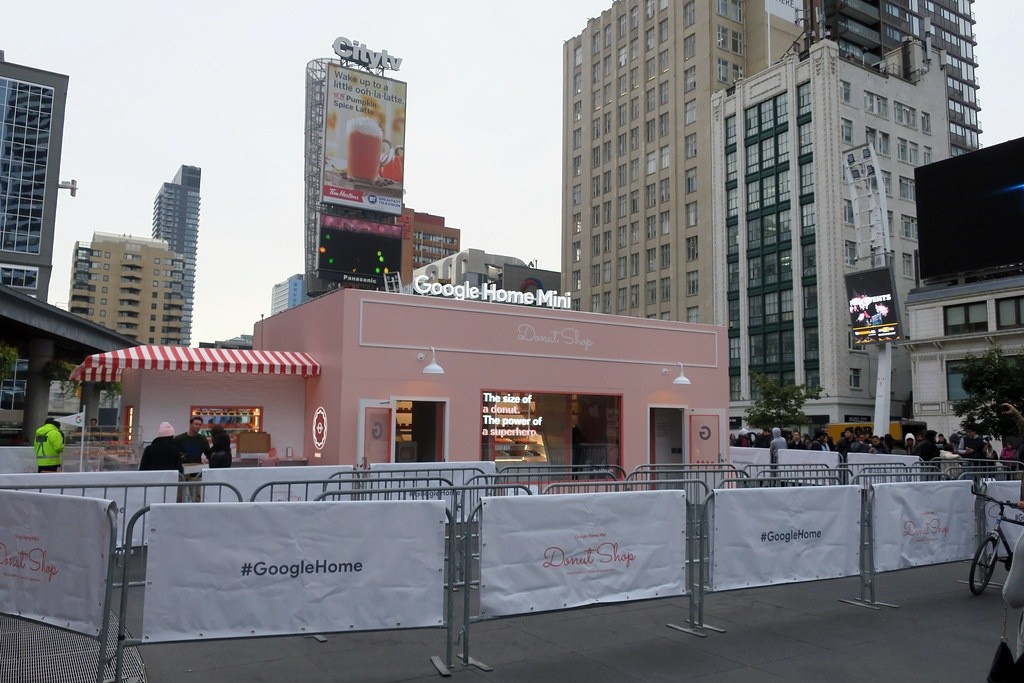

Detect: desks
97;452;139;472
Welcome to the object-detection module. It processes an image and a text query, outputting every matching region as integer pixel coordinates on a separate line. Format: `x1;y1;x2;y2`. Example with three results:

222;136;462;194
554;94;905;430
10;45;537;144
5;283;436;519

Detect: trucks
826;418;929;446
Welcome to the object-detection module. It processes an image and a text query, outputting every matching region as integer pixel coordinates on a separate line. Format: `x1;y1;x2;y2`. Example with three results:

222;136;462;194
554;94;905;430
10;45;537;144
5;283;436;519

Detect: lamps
663;362;691;384
417;347;444;374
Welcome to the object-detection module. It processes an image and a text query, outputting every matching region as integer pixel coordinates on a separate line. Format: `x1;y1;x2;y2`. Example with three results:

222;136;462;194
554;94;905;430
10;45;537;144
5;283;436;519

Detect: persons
939;444;961;478
770;427;788;457
1018;441;1024;470
572;415;586;480
174;415;211;477
958;423;986;480
984;444;998;477
140;422;184;474
207;425;232;468
739;429;750;447
34;417;64;473
916;430;940;466
1000;439;1017;480
754;428;774;448
949;431;962;450
87;419;103;441
1002;529;1024;660
938;433;947;446
787;427;918;463
915;432;922;441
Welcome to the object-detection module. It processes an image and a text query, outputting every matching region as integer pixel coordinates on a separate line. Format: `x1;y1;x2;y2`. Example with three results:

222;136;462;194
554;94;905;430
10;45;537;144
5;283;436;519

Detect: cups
347;117;394;184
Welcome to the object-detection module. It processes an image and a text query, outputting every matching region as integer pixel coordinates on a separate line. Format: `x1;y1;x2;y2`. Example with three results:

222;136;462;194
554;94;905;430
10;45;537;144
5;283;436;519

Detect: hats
740;429;749;436
925;430;937;439
158;422;174;437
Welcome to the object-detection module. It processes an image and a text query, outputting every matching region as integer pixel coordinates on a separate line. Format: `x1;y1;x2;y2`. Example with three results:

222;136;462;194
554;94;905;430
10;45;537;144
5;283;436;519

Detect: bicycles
967;484;1024;594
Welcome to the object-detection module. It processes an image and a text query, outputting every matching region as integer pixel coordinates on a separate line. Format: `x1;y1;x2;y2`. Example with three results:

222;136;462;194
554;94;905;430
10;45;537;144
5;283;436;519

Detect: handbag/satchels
987;642;1024;683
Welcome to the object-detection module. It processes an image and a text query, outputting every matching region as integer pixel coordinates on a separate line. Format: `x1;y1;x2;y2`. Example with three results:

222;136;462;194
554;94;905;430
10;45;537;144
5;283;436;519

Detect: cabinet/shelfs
496;397;546;459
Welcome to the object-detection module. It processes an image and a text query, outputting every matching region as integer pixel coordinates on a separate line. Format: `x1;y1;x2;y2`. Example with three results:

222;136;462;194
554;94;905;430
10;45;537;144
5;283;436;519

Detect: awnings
70;344;321;382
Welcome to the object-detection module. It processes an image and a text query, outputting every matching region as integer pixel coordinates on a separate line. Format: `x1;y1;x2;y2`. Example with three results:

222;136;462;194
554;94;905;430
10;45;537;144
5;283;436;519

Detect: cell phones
997;405;1009;412
